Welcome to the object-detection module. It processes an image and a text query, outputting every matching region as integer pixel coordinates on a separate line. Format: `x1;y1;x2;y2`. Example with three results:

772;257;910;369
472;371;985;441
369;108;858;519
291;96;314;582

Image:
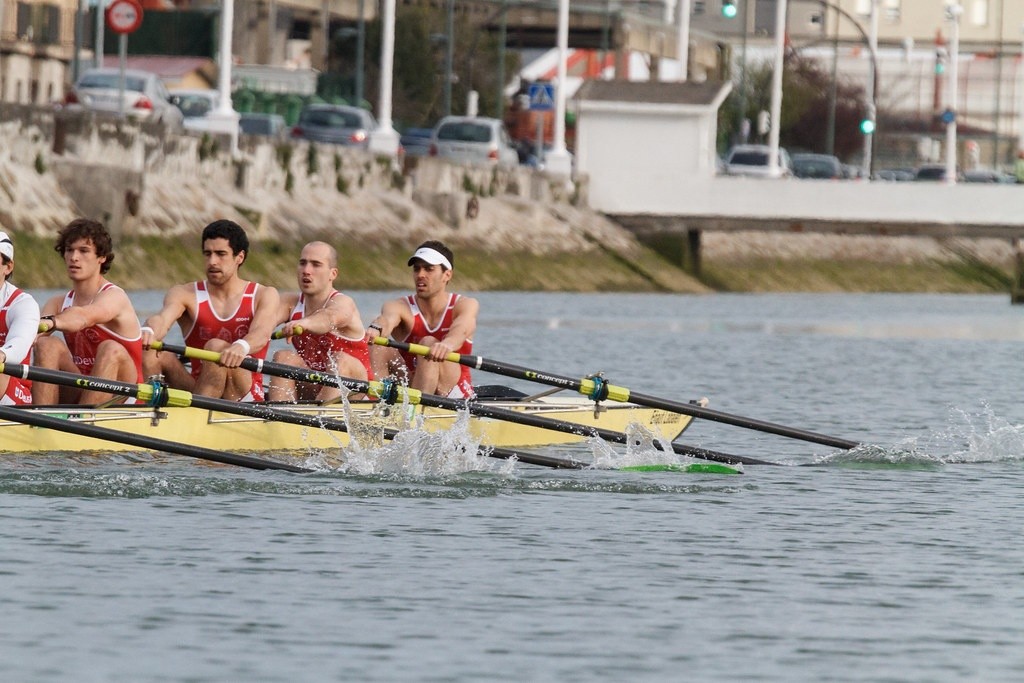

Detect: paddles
374;337;861;451
0;404;317;474
0;363;744;476
151;340;788;466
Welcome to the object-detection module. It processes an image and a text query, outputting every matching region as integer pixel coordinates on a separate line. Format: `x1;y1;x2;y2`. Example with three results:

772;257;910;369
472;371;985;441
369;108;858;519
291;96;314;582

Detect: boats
0;394;709;455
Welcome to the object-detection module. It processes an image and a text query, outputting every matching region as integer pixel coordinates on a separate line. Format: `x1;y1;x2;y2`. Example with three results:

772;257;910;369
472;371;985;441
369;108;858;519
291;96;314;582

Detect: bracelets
233;339;250;355
369;325;382;337
141;327;154;335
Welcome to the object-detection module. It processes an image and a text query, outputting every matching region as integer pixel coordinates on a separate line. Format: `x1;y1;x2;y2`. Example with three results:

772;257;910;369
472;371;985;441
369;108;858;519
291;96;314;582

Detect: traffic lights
722;4;738;18
861;120;876;135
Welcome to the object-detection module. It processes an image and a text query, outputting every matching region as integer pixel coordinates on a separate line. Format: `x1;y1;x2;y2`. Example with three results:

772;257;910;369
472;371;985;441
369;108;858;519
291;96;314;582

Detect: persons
0;231;41;405
268;240;373;402
364;240;479;400
30;218;145;405
141;219;281;402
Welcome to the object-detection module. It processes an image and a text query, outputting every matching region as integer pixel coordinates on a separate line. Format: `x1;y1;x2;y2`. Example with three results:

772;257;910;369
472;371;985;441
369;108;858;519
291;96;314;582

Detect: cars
240;105;378;147
716;143;1019;183
63;68;184;135
400;115;574;171
170;89;243;135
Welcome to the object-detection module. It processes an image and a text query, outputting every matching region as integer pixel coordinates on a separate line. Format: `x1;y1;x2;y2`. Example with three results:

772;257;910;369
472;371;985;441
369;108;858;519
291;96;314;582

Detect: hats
407;248;452;271
0;231;14;260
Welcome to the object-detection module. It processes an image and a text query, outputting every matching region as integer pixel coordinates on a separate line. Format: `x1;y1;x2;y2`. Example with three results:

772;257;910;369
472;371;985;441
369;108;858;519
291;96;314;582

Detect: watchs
40;316;56;333
